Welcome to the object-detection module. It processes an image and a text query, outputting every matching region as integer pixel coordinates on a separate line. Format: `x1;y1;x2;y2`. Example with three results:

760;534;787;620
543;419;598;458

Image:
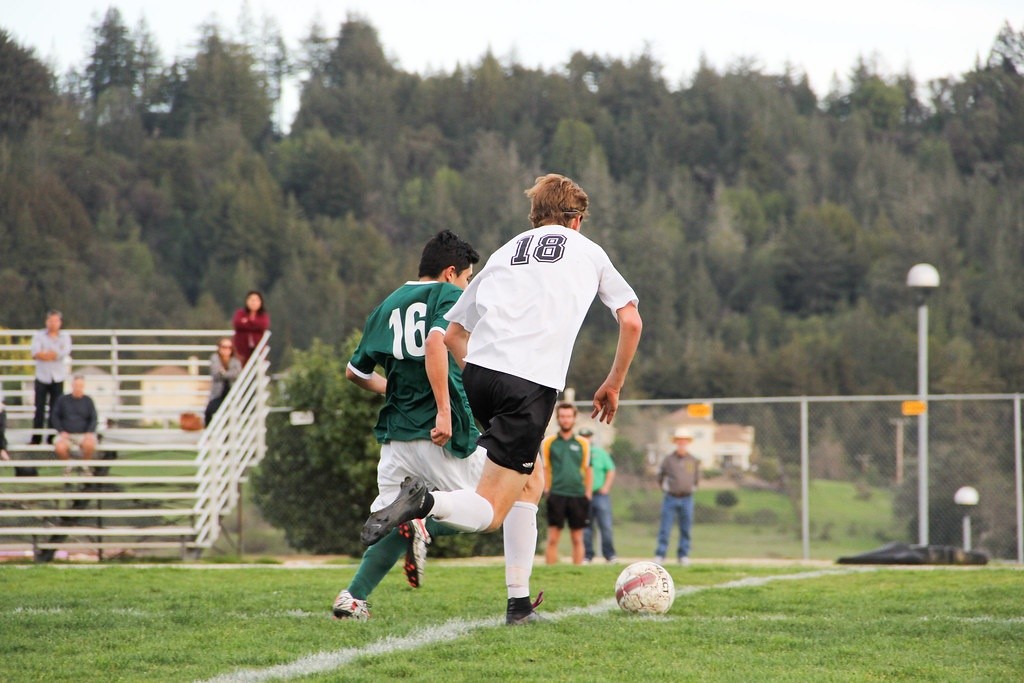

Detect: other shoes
679;556;692;567
583;558;593;566
607;556;620;565
652;555;664;564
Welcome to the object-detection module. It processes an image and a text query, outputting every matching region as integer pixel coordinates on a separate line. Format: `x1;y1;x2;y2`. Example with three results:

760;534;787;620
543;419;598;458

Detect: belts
667;491;693;498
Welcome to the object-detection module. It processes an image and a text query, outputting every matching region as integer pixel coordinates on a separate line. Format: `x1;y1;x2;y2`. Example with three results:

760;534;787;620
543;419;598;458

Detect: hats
579;428;594;436
671;428;695;444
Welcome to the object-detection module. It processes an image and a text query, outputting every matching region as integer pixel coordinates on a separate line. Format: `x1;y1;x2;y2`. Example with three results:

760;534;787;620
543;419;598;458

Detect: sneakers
62;466;73;477
504;590;555;626
399;517;432;588
333;590;372;621
78;465;94;476
360;475;434;547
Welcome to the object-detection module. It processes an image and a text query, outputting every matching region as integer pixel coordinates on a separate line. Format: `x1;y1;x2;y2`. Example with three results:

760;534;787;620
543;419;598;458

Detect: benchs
0;371;272;560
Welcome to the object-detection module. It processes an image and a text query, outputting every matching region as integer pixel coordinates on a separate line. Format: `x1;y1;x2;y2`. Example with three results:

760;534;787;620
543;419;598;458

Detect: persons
202;338;243;431
29;310;72;448
313;172;703;627
0;402;13;465
230;291;271;370
52;376;98;478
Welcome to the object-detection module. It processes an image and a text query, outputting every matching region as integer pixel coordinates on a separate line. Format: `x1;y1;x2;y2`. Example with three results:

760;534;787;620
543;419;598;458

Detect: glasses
219;346;234;350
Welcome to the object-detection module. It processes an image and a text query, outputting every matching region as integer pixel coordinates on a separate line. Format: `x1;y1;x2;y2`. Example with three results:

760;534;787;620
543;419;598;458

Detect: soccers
614;561;676;616
